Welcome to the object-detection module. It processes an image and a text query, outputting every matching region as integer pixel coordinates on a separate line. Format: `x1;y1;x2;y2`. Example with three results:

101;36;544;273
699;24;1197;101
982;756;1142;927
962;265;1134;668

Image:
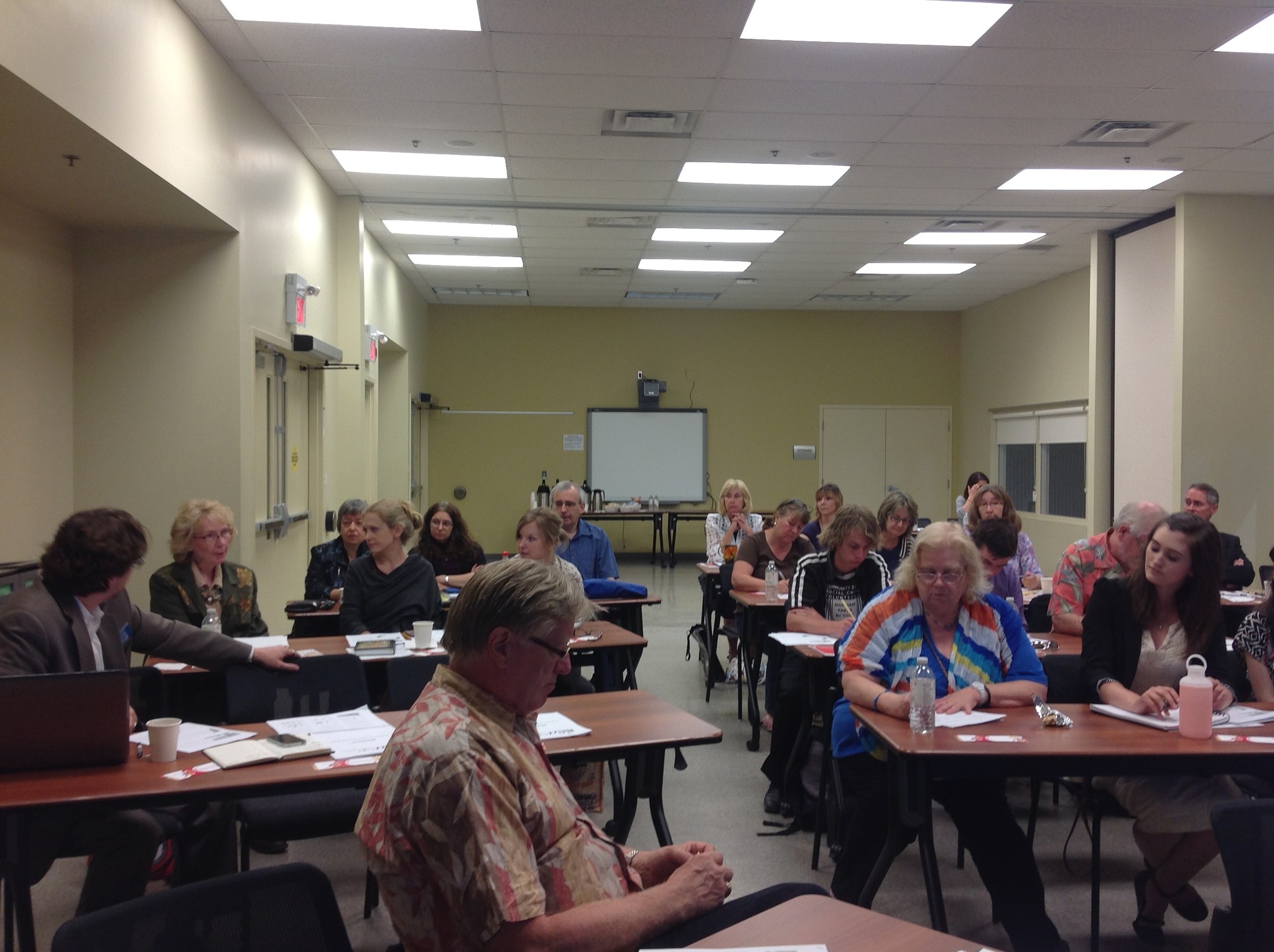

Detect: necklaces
1150;605;1176;629
923;608;959;631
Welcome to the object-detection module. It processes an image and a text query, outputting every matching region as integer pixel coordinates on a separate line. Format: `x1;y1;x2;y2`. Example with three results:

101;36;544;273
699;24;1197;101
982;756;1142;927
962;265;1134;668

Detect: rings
291;649;295;655
724;881;732;897
133;722;137;728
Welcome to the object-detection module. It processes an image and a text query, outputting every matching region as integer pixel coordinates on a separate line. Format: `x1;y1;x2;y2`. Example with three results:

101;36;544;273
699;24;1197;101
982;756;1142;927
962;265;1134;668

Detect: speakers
644;381;660;397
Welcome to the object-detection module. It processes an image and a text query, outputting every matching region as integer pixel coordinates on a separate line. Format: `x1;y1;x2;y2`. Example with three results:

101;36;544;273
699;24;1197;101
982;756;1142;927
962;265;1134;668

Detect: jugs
537;479;605;512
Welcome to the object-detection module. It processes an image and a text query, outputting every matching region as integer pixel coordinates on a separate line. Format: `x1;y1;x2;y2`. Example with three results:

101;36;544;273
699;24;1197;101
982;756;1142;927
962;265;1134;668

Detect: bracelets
983;685;991;707
873;689;891;713
625;849;640;867
444;574;449;586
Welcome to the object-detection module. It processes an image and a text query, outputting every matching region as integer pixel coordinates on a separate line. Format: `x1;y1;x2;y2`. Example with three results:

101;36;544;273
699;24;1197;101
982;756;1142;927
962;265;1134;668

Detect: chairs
51;860;354;952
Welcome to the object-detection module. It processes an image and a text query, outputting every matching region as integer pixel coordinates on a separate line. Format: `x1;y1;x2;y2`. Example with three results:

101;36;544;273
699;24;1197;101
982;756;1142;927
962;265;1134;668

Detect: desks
667;507;775;569
0;684;723;952
128;618;659;873
691;896;1002;952
694;558;1274;934
585;507;668;570
288;569;666;682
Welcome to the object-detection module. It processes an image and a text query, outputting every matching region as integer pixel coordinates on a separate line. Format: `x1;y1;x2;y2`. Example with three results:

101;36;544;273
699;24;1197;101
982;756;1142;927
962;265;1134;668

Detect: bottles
502;552;509;560
1179;654;1213;738
1006;597;1019;614
765;560;778;601
201;608;222;634
909;657;934;735
649;495;659;511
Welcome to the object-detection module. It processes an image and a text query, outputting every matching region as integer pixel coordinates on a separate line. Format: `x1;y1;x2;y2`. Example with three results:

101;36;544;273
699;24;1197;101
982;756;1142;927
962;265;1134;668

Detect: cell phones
268;734;305;747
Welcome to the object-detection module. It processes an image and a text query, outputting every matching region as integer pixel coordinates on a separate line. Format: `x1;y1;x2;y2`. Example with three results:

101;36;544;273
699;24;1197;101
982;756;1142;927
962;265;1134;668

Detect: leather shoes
763;781;783;813
252;841;288;854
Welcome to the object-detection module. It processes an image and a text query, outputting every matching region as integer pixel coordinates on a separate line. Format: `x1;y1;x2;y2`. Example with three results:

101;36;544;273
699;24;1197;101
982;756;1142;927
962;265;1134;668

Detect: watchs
971;680;986;705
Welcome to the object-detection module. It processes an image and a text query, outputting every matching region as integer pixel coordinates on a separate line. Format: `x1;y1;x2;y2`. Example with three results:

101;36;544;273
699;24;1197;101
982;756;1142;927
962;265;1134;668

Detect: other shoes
1132;869;1168;946
1152;865;1208;922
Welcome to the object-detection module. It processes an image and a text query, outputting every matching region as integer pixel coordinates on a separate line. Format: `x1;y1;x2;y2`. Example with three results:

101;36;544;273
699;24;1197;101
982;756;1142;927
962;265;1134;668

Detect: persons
543;480;621;685
1183;482;1255;636
1229;573;1274;798
505;508;599;698
338;498;445;706
347;555;835;950
142;497;271;667
827;520;1074;952
290;498;372;637
705;468;1045;819
1061;511;1251;946
406;502;487;594
0;501;302;918
1045;499;1169;638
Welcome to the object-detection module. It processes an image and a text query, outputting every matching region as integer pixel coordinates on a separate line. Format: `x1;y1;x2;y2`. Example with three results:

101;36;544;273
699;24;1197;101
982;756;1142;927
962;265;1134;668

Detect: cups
340;587;343;604
413;620;434;648
146;717;182;762
1041;577;1053;595
913;524;918;531
1263;580;1272;599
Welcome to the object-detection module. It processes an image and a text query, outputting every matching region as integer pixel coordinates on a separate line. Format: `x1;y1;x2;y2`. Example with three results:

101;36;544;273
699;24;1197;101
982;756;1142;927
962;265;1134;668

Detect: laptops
0;669;128;778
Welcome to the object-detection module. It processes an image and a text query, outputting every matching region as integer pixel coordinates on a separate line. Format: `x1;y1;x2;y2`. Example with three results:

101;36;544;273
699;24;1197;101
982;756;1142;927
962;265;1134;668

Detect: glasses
1131;531;1146;550
915;565;966;583
890;513;912;526
573;627;603;642
193;528;233;542
513;627;570;659
133;557;145;570
776;499;813;514
